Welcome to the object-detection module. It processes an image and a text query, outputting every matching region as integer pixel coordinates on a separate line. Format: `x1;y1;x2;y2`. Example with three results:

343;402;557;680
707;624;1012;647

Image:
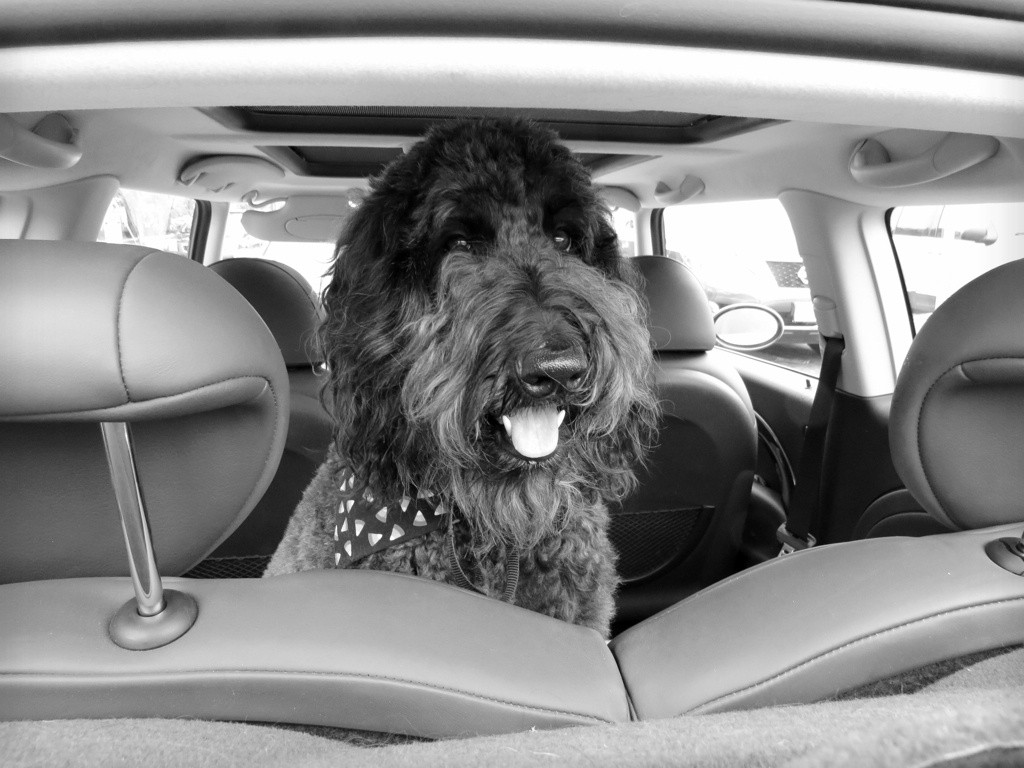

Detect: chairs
0;239;1024;741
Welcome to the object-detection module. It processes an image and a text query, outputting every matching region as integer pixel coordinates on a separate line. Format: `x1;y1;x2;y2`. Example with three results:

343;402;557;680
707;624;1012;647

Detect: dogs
262;115;663;642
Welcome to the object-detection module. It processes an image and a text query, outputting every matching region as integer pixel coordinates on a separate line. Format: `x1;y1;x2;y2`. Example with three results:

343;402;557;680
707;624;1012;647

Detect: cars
99;187;184;256
